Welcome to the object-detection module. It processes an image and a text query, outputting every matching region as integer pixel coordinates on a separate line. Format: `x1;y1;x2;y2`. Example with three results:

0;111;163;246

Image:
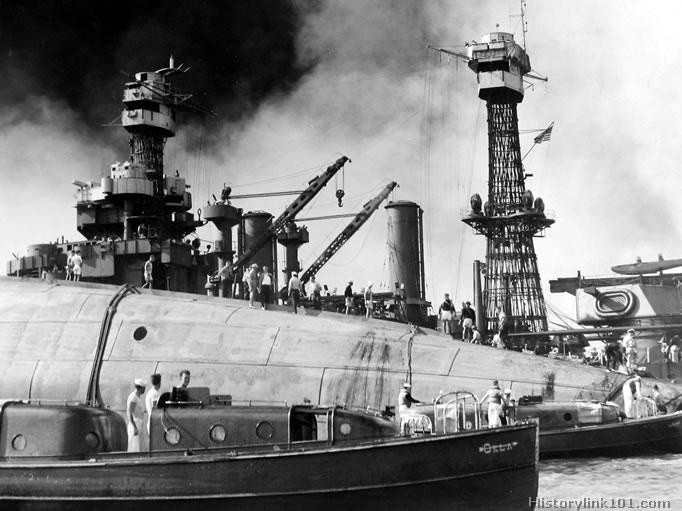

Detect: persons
125;378;149;453
178;370;191;389
143;255;157;289
72;250;83;282
147;372;163;435
602;326;680;385
500;390;511;426
66;251;75;281
364;281;374;319
439;294;454;334
652;385;667;416
398;384;421;437
495;306;509;349
240;261;332;315
458;301;475;342
470;325;481;344
478;380;507;427
343;281;354;315
622;373;644;419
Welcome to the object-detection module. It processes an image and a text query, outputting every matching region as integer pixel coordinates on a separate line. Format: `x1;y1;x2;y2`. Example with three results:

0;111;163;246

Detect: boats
0;375;552;511
2;54;680;412
394;378;682;464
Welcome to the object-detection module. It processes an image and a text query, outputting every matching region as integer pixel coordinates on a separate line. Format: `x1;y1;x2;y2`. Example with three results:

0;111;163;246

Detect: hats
290;270;297;276
490;380;499;389
250;263;259;268
402;382;411;388
134;377;147;387
626;328;635;332
